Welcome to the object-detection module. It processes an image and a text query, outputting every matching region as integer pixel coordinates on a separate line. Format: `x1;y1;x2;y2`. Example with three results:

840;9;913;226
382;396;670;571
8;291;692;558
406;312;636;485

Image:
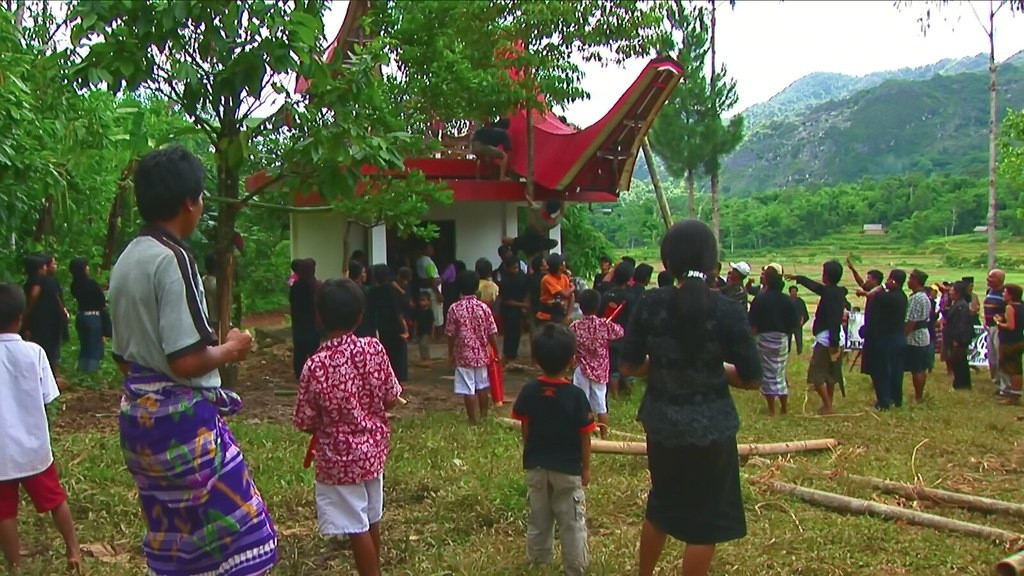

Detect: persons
509;320;597;576
617;218;764;576
68;257;112;375
290;275;402;576
107;145;278;575
287;198;1024;438
472;117;512;181
0;283;85;574
19;252;70;389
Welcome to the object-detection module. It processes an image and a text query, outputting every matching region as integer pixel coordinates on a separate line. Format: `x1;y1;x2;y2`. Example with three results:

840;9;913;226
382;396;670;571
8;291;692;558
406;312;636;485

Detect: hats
934;281;952;290
764;263;784;275
729;261;750;276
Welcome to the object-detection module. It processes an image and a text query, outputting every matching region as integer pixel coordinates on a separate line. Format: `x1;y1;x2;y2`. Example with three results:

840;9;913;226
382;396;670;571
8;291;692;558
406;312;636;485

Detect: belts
78;310;100;316
